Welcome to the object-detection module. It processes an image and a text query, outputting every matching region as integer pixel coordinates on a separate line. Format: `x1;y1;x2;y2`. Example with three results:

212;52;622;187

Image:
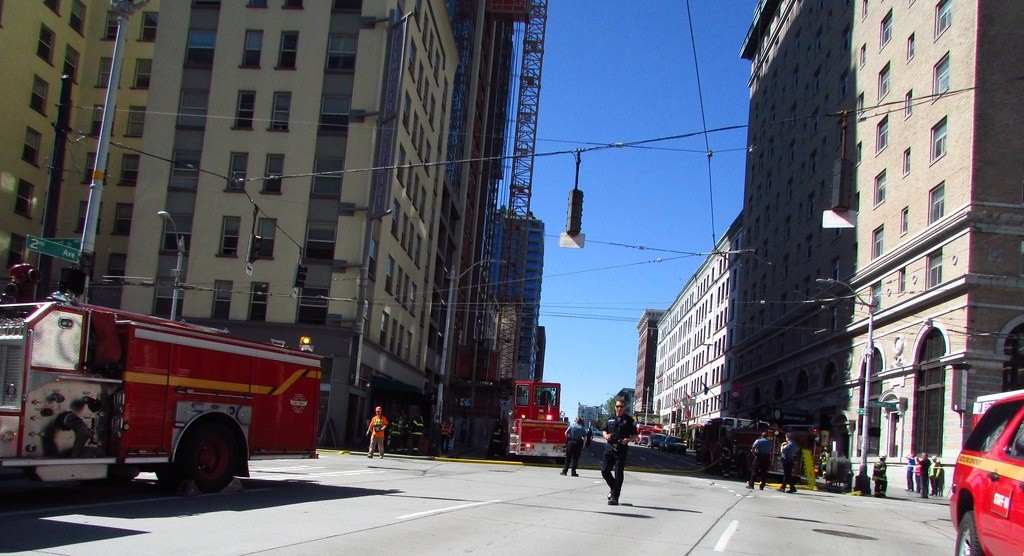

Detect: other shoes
759;486;763;490
380;456;383;459
560;471;567;476
776;487;784;492
571;473;579;476
368;454;373;458
745;485;754;489
787;488;797;493
608;492;618;505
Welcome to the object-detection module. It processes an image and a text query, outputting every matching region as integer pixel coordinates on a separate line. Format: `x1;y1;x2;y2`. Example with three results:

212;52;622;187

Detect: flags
673;385;710;408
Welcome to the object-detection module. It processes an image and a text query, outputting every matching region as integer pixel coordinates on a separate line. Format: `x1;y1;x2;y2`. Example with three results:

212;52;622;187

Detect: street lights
430;257;508;453
815;278;874;496
157;210;185;321
630;382;650;426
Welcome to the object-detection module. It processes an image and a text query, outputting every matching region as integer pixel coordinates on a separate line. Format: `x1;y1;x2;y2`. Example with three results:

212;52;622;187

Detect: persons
585;428;593;448
776;432;798;493
820;446;943;497
602;399;639;505
560;418;587;477
366;406;454;458
485;418;508;459
746;432;771;490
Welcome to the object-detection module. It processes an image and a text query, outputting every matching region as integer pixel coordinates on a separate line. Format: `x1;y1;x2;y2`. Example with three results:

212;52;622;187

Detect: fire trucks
0;300;325;498
696;417;829;486
507;379;569;465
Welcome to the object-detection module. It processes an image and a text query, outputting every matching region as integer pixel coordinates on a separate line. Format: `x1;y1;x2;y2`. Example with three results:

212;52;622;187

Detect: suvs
634;424;666;449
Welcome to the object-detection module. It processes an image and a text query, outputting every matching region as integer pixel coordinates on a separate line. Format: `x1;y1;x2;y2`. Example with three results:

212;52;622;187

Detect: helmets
376;407;382;412
879;453;887;459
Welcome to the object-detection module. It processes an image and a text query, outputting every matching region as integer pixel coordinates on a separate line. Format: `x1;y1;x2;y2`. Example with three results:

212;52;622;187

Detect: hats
931;455;937;459
786;432;796;439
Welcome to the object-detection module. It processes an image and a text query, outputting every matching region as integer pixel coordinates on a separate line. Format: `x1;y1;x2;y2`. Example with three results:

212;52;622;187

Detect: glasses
616;405;624;408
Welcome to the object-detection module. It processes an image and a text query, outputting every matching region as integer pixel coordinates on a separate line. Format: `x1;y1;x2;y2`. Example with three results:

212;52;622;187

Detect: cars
592;427;603;436
949;393;1024;556
663;436;686;454
